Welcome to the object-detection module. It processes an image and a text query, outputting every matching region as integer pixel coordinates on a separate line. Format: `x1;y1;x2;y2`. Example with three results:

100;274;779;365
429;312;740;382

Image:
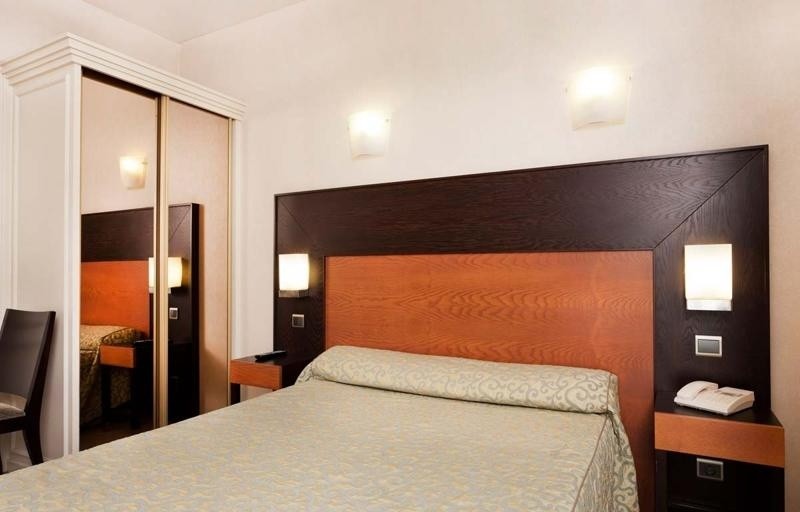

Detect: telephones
674;380;755;417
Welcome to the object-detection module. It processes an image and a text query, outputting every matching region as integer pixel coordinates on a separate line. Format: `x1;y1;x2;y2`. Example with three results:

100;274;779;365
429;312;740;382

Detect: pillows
295;344;618;415
80;325;136;351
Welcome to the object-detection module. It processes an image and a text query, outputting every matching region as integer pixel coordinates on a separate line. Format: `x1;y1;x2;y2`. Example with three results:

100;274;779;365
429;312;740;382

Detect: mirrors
80;65;231;453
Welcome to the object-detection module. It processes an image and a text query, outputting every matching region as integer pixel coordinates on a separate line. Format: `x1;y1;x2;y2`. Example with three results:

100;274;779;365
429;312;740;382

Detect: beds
0;345;640;512
80;324;136;428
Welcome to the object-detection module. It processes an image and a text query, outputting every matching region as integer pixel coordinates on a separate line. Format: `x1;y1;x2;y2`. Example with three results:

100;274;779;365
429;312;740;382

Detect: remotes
134;339;153;346
255;350;288;361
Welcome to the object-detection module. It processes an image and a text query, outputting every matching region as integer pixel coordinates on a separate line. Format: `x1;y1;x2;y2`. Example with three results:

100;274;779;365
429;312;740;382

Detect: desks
230;352;312;406
98;343;153;425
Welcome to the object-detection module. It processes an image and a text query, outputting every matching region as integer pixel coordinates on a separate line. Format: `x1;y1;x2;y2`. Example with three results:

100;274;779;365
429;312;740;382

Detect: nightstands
654;403;785;512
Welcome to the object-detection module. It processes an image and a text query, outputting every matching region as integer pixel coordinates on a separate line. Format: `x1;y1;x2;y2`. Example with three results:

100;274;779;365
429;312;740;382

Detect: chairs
1;308;56;475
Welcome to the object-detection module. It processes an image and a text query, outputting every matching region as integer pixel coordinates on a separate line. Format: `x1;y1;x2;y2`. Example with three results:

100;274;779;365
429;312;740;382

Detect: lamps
278;253;310;299
682;244;734;312
349;108;393;158
571;55;632;132
120;153;147;190
149;256;183;289
